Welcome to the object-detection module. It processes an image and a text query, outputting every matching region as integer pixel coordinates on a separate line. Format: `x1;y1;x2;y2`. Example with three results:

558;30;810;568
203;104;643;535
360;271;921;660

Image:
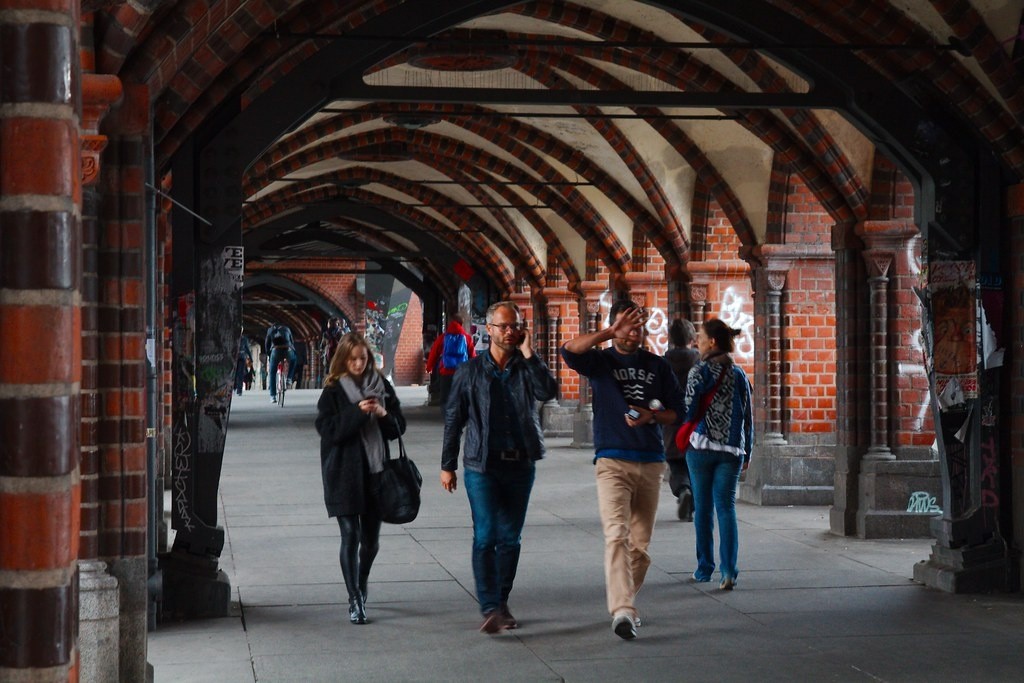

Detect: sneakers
719;576;735;591
611;615;642;639
479;604;516;632
692;572;697;580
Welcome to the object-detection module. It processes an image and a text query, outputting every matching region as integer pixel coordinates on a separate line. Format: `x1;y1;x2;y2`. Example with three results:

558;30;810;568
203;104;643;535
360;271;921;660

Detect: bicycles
274;356;291;409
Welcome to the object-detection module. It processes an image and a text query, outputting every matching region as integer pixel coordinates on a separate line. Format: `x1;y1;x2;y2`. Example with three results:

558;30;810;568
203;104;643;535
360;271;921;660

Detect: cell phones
519;334;526;344
365;395;376;402
628;409;640;420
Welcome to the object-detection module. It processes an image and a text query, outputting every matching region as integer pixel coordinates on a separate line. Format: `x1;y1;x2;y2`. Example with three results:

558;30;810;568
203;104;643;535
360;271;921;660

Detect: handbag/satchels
675;413;702;455
381;416;422;525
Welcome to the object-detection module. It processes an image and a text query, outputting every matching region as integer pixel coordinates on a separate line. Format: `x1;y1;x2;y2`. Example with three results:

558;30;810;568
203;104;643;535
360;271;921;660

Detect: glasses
489;322;522;330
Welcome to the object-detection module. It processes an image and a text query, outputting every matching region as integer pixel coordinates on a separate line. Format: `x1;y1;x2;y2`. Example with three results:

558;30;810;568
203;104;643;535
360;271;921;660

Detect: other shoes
285;378;292;389
678;489;694;522
273;396;277;404
349;589;368;624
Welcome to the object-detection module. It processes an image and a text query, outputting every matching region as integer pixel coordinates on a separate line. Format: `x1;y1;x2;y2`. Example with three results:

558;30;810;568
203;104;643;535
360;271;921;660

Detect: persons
264;323;298;403
236;325;257;396
314;329;407;623
425;315;478;419
319;317;352;376
661;316;754;590
561;299;686;640
441;300;558;635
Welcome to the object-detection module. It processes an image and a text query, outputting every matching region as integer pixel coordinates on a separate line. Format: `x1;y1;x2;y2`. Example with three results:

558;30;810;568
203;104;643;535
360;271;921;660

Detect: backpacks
271;329;290;345
325;331;340;362
440;333;469;370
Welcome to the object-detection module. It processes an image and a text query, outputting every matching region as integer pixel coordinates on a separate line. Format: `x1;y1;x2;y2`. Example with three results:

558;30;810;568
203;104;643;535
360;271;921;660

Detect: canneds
650;399;667;413
